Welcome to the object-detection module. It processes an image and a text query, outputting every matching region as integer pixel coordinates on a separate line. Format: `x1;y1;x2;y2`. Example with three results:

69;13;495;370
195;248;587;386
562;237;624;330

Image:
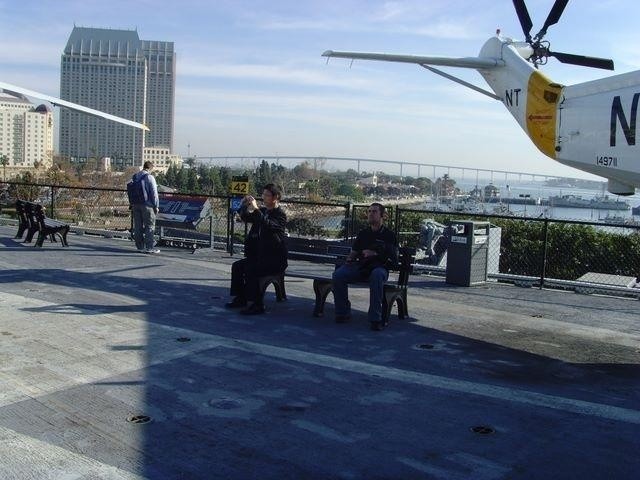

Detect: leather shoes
240;304;262;314
371;321;381;331
226;298;243;308
336;316;351;323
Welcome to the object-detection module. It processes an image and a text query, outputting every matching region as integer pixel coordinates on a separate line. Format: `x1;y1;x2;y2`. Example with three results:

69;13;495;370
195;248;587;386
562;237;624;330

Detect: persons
224;183;287;316
332;202;400;333
129;161;161;255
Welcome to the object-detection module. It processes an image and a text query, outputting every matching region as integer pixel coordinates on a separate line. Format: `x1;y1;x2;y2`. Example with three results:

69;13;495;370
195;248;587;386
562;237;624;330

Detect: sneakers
137;248;160;253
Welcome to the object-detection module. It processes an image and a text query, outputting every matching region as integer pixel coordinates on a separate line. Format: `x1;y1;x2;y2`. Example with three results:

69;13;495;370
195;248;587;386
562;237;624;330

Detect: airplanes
322;2;640;196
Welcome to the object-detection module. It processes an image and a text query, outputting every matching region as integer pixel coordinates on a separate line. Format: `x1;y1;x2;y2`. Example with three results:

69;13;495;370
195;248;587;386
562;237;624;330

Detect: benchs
230;237;415;326
15;198;70;248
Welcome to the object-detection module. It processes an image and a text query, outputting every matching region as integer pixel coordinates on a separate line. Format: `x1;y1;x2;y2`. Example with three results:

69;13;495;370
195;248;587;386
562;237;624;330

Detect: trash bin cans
446;221;502;286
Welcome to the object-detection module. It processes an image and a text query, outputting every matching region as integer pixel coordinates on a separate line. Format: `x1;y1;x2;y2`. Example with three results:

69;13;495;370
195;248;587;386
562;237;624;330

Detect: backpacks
127;173;150;203
